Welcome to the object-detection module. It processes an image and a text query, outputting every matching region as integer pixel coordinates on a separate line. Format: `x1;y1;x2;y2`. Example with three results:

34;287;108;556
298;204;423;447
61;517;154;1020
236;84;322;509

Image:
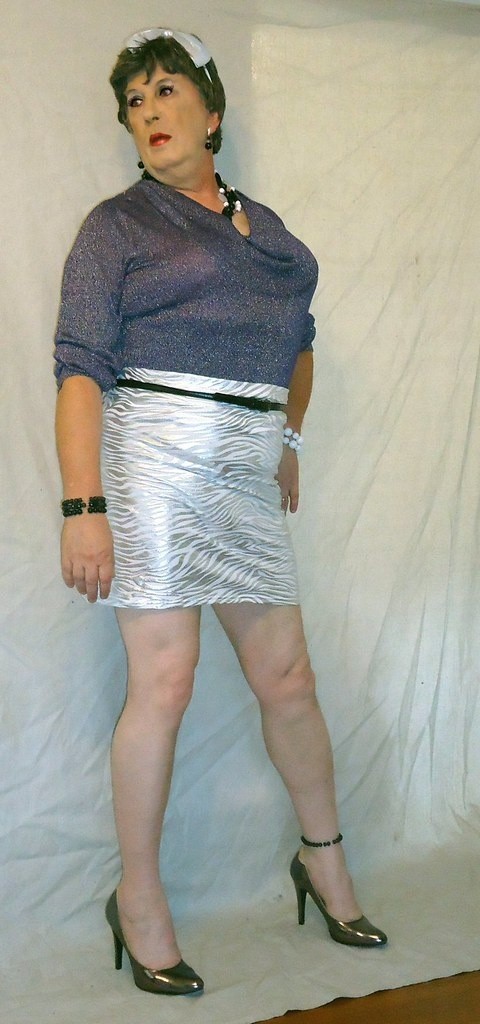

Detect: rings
281;497;288;501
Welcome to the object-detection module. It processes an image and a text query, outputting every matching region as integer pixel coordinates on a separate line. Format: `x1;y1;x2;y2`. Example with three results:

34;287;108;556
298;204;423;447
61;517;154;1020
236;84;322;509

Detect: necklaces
141;169;241;222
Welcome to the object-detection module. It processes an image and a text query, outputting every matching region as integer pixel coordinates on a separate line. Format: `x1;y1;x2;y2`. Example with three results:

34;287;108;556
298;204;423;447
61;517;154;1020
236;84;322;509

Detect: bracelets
283;428;304;451
61;496;108;518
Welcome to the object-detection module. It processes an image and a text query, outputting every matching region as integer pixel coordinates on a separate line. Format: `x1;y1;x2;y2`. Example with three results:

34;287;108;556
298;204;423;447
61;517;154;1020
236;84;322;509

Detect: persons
53;28;387;995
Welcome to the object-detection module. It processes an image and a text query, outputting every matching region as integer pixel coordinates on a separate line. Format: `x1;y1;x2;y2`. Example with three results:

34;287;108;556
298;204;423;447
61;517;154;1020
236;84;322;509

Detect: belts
116;378;288;412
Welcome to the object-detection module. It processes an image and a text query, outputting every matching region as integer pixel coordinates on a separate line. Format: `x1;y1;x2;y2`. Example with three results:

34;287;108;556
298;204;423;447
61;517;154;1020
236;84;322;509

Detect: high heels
290;845;388;948
105;889;205;995
